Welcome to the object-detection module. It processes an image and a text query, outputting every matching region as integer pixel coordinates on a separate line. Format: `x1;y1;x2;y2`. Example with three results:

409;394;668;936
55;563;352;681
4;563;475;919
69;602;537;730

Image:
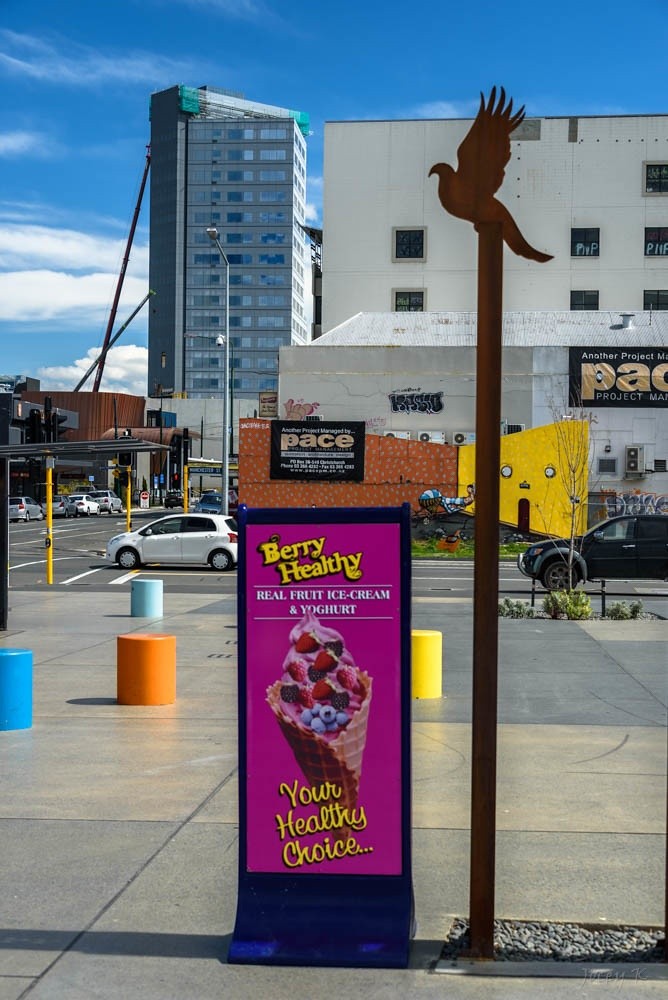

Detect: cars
9;496;45;521
69;495;101;517
193;493;222;517
515;514;668;592
105;512;238;571
163;491;189;509
38;494;79;519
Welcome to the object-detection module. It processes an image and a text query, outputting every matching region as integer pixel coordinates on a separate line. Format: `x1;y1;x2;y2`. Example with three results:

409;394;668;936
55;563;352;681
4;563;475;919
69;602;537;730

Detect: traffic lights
170;434;182;465
23;410;42;444
117;436;133;468
118;471;130;488
52;412;70;442
172;472;181;489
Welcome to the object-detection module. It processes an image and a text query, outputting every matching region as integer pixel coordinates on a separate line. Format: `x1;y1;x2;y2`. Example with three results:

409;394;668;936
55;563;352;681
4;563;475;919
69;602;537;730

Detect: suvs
86;490;124;514
73;485;96;496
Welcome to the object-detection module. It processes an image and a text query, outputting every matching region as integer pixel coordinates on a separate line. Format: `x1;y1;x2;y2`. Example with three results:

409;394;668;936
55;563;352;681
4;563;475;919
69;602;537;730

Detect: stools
0;647;34;730
410;631;442;697
132;579;164;615
117;635;177;705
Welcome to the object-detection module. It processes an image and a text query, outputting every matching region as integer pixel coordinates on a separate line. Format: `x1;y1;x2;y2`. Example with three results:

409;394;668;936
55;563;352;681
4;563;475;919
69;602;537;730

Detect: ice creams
266;606;373;856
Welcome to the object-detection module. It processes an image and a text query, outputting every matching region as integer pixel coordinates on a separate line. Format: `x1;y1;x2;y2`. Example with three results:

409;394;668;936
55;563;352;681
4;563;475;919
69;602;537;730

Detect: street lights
205;225;231;518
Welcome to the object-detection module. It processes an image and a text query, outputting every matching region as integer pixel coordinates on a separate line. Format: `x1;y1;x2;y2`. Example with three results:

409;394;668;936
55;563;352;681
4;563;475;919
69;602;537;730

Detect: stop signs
141;492;149;500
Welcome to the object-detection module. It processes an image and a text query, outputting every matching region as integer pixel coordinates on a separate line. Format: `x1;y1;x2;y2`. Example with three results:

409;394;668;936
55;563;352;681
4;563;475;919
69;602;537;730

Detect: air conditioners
384;431;411;440
418;431;446;444
627;446;642;473
503;424;526;435
453;432;476;445
305;415;324;421
